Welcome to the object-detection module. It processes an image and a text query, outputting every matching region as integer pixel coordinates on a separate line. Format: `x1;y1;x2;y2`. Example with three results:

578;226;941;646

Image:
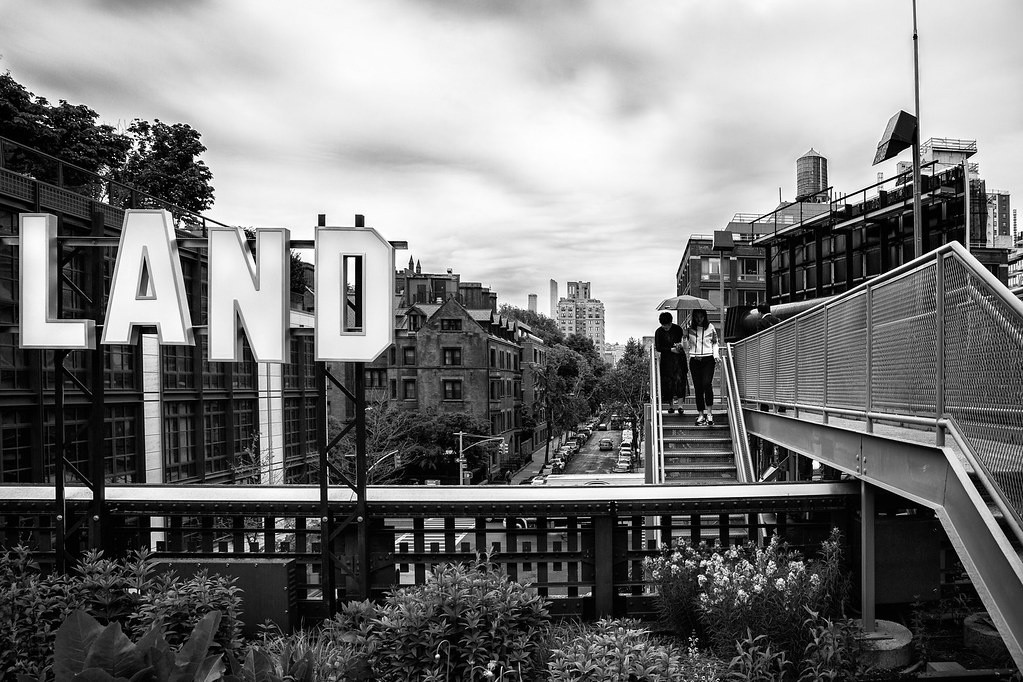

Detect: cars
527;415;634;484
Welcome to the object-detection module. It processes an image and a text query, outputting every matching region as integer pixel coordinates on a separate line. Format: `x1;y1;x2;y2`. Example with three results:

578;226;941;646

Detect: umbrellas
656;295;717;328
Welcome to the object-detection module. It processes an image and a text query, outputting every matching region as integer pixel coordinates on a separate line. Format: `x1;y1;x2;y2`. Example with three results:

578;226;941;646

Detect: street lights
871;0;924;316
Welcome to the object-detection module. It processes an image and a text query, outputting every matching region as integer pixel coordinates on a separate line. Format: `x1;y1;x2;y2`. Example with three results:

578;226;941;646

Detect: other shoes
668;408;675;413
706;413;714;426
694;416;706;426
678;407;684;414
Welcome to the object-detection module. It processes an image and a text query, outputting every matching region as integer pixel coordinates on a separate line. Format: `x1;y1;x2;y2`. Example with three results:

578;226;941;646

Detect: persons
655;312;690;414
505;469;513;485
756;301;788;413
683;309;722;426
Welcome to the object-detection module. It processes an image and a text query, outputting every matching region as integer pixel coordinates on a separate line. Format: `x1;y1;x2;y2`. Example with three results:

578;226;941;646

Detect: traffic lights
503;443;509;455
498;442;503;455
